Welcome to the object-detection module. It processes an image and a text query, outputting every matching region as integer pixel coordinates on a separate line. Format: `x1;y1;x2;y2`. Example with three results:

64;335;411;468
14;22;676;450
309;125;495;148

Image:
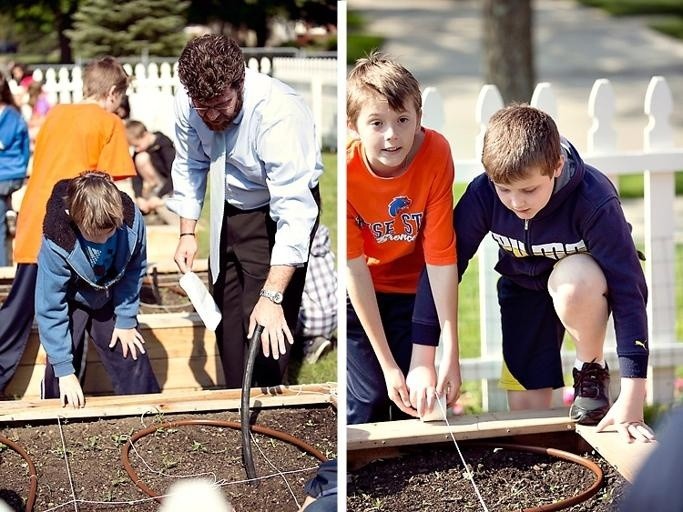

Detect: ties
210;132;227;286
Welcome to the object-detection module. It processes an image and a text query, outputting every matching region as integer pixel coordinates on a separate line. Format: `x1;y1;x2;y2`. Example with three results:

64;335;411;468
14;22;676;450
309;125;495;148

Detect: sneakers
308;336;331;366
570;360;610;423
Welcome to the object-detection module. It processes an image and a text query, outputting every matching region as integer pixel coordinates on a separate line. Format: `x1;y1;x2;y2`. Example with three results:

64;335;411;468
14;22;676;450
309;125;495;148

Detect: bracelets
179;232;196;238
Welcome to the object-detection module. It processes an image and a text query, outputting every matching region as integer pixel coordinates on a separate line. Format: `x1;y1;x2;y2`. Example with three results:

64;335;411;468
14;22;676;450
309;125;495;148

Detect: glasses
187;96;236;112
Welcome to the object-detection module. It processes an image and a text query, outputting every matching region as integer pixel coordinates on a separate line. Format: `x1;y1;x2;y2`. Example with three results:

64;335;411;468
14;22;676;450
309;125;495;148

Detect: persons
406;104;657;448
0;52;177;411
348;52;463;423
163;29;327;389
293;457;338;512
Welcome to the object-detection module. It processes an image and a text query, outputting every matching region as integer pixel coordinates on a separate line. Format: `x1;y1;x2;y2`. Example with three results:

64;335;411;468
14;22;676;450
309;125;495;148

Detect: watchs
259;289;283;304
